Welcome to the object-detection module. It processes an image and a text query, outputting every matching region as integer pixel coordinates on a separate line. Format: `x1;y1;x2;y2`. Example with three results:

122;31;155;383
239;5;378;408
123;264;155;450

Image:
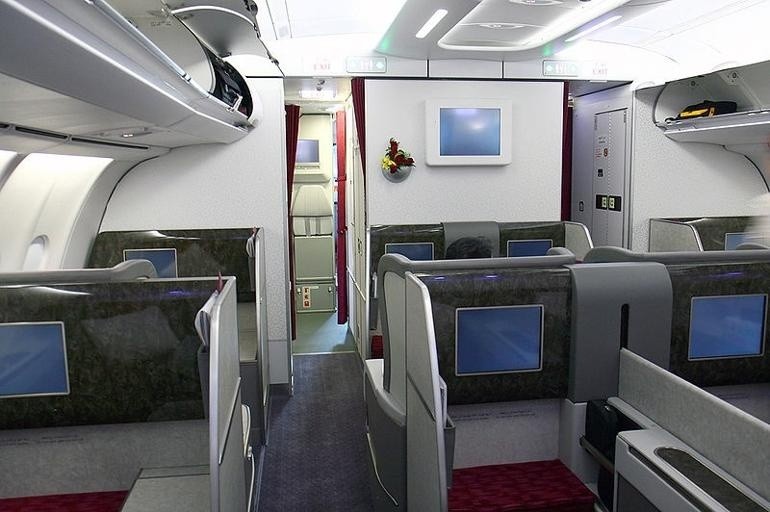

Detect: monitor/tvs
384;241;435;261
296;139;320;167
0;321;70;398
506;238;553;257
425;97;513;166
725;232;746;251
687;293;768;362
122;247;178;278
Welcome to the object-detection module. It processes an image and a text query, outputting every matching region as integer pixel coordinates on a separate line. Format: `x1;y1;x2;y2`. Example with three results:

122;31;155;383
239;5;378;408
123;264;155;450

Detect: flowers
380;139;414;173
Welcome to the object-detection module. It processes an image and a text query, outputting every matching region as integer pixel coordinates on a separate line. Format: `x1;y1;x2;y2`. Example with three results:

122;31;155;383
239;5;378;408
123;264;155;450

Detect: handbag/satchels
675;100;737;120
209;54;244;112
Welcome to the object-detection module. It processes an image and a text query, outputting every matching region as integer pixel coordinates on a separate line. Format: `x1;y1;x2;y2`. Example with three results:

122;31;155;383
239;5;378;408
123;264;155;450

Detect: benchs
85;227;270;398
1;257;264;510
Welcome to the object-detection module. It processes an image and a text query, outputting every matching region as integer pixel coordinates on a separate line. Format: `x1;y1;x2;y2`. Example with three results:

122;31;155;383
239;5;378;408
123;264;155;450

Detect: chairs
371;216;769;511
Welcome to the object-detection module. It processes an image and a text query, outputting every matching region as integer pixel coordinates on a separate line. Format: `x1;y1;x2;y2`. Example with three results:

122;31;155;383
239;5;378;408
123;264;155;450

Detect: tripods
454;303;544;376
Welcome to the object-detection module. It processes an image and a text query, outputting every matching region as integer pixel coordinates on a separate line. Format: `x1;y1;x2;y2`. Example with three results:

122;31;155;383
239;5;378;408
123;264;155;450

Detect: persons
445;237;492;259
